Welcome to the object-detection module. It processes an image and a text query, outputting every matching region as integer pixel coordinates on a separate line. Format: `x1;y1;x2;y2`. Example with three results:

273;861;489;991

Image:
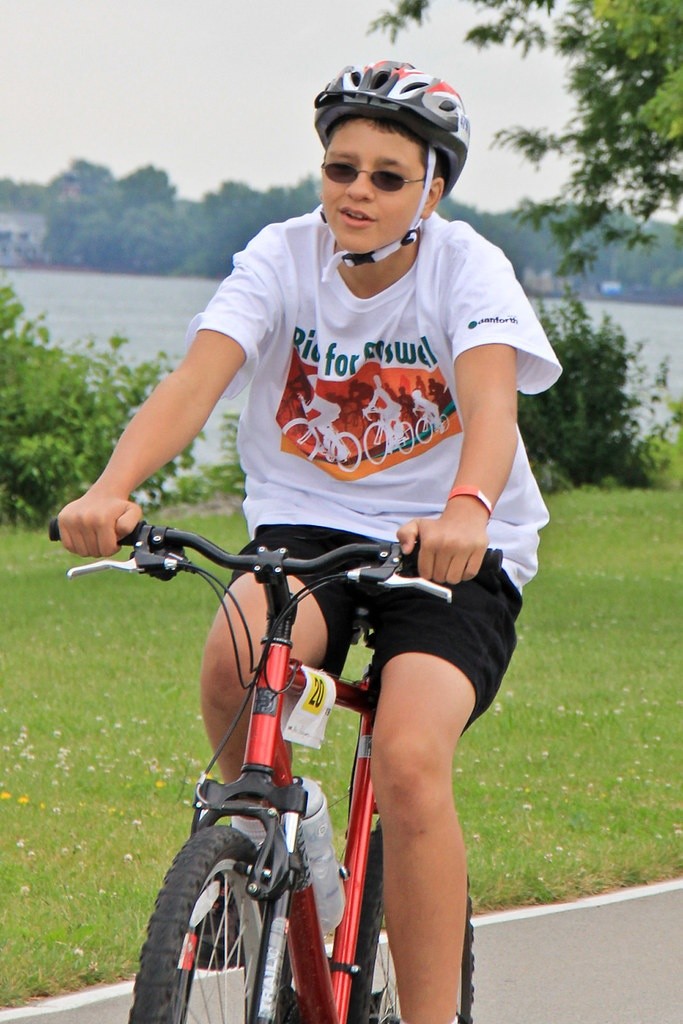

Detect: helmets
313;59;471;201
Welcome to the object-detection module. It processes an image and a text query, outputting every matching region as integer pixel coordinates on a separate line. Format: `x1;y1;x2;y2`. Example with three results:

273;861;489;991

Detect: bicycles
46;515;478;1024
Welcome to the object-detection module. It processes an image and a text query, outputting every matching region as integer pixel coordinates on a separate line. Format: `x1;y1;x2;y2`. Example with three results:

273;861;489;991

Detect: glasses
321;162;425;193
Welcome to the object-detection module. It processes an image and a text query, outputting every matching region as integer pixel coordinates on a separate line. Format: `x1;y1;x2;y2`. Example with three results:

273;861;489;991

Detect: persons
52;53;551;1022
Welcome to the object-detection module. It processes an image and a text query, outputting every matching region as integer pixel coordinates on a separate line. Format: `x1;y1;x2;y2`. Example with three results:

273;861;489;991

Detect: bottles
294;776;346;931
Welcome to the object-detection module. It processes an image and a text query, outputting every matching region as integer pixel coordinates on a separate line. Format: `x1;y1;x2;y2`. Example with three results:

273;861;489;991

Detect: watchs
444;486;494;518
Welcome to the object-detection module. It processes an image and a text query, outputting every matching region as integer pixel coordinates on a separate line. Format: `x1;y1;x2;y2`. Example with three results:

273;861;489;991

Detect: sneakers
189;824;273;971
